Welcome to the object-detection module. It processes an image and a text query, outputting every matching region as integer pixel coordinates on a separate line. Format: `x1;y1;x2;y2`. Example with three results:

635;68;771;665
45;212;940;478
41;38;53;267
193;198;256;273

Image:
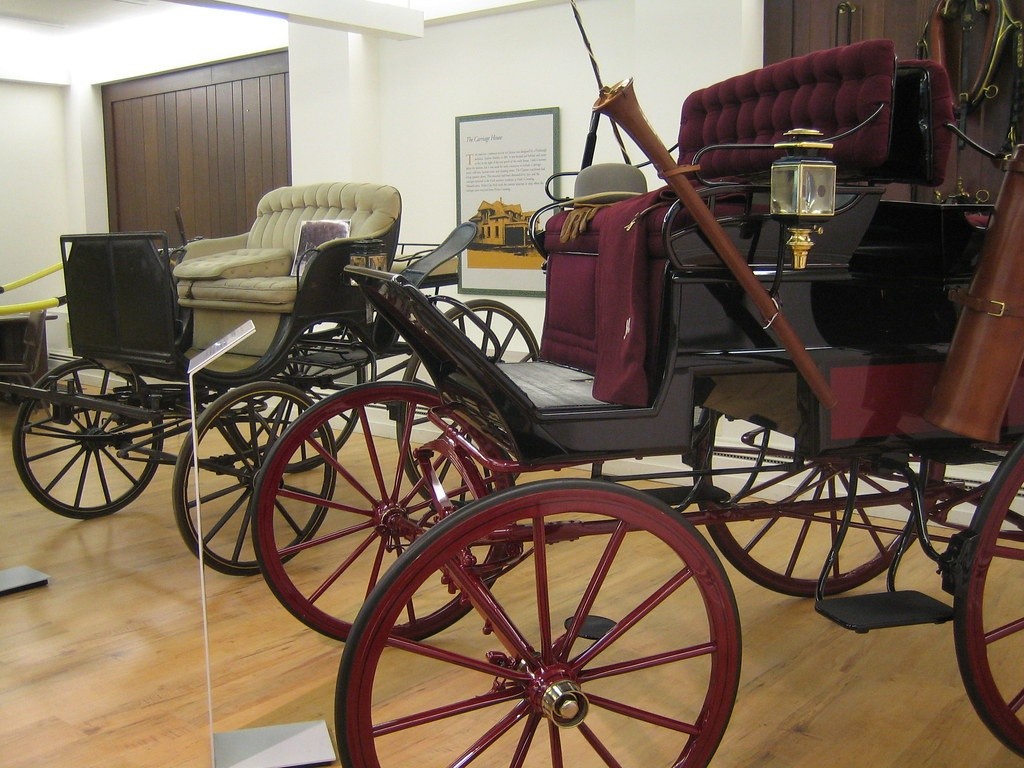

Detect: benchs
528;37;996;373
173;181;402;314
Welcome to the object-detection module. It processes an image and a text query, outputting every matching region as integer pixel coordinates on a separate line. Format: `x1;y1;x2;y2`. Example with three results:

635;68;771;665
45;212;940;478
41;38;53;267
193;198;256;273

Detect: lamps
770;128;837;271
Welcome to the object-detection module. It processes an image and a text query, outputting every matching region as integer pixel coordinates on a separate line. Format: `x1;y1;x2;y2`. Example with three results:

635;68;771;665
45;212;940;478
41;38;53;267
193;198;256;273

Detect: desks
0;312;58;409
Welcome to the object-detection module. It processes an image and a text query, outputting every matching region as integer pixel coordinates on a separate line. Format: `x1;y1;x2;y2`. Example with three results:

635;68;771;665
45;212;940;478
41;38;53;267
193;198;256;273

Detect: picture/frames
455;107;561;297
